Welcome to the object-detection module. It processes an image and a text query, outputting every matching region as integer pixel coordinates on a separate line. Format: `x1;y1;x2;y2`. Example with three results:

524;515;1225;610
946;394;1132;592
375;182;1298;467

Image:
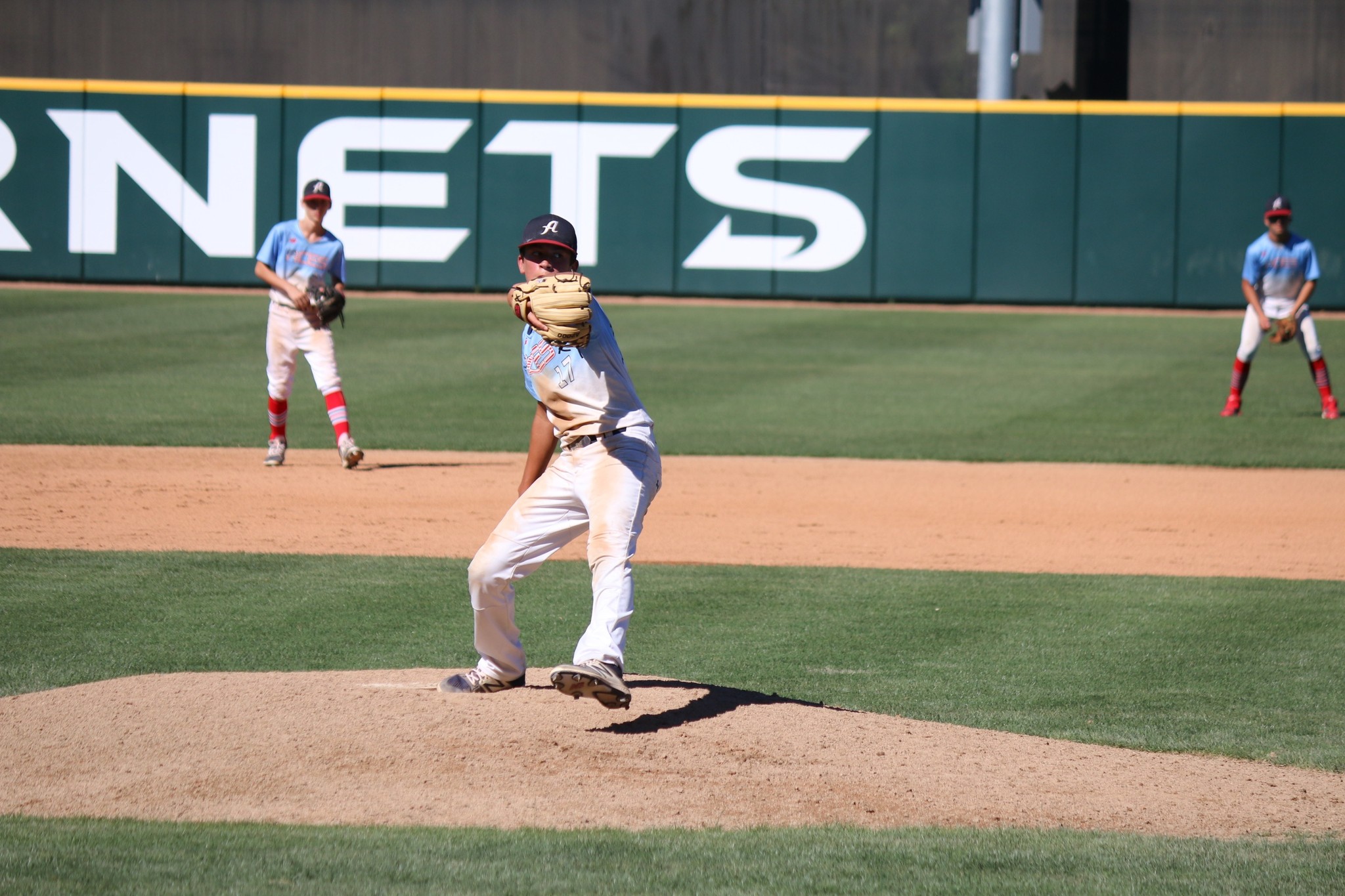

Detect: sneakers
1222;401;1241;418
438;663;526;695
264;437;283;465
1321;394;1340;420
338;444;362;469
550;655;631;709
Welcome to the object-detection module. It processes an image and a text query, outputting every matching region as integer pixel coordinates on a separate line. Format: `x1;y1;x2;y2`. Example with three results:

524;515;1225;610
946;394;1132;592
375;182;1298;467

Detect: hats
516;215;578;258
304;180;330;201
1266;194;1292;219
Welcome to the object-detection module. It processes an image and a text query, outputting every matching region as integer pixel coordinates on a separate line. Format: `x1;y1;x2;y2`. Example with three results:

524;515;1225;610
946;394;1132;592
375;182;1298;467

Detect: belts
567;427;627;451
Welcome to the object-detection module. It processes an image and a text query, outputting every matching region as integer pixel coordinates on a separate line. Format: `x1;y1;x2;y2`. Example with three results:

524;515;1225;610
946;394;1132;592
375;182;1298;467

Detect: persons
255;178;365;468
1222;195;1338;419
437;214;662;712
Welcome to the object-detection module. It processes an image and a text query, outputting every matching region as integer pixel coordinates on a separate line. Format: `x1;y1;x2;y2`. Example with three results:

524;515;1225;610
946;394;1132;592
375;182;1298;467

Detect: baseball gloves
1270;317;1296;343
511;268;593;359
315;286;346;329
